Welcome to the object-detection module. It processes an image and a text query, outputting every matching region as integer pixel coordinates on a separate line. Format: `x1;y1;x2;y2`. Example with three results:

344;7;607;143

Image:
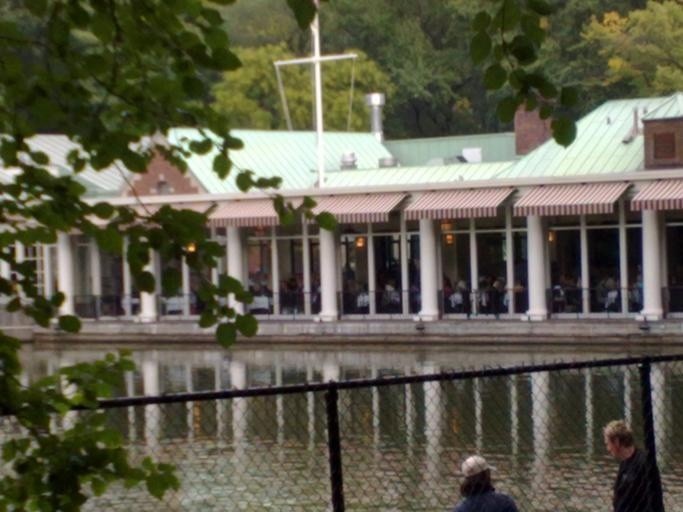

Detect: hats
461;455;497;478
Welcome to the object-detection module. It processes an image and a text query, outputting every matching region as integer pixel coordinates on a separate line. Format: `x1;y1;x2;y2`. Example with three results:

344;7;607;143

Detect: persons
450;455;517;511
602;418;658;511
